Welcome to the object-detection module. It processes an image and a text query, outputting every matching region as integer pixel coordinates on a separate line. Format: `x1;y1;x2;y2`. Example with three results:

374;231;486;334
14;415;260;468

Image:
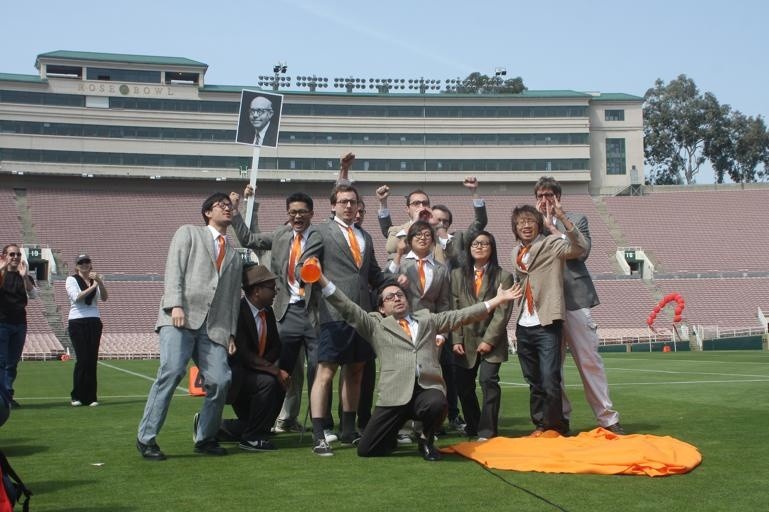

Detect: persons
338;153;408;432
386;191;457;269
229;190;341;447
535;176;624;436
137;192;242;462
242;96;278;146
304;257;523;461
65;254;108;407
193;264;290;449
383;220;452;444
0;244;35;403
450;232;515;442
377;175;488;432
509;195;588;437
295;183;409;458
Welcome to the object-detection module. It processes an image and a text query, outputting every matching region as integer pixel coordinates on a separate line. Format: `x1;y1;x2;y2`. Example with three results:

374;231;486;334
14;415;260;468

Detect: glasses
335;199;357;205
381;289;404;305
287;210;309;217
10;252;21;258
415;231;432;240
406;200;429;207
212;201;233;210
357;209;366;215
537;193;554;199
76;259;91;265
471;240;490;248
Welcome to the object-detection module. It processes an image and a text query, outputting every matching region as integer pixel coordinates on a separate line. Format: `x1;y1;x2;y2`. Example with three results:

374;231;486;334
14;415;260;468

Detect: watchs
434;224;449;230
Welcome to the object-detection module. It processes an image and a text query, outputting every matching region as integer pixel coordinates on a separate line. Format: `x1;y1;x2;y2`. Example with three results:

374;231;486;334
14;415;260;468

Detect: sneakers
70;399;81;406
563;421;572;437
447;417;467;431
270;419;309;433
603;422;625;436
238;438;279;451
398;433;412;444
193;412;199;441
341;433;362;446
323;428;338;444
312;439;335;457
90;401;98;406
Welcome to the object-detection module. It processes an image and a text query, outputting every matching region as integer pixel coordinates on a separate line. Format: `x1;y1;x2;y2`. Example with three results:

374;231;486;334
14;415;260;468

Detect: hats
75;252;92;264
242;264;278;287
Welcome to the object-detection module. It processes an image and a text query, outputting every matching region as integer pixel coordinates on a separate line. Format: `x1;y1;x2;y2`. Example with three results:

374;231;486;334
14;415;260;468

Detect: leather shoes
521;428;544;440
9;398;22;410
541;430;557;439
416;437;442;461
193;440;229;456
136;438;167;461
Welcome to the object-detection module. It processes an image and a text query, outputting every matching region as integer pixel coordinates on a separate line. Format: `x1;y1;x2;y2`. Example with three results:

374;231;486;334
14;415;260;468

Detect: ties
400;319;411;338
475;271;484;299
257;311;268;355
289;234;302;284
417;260;426;294
516;245;533;315
346;226;362;269
217;237;227;271
